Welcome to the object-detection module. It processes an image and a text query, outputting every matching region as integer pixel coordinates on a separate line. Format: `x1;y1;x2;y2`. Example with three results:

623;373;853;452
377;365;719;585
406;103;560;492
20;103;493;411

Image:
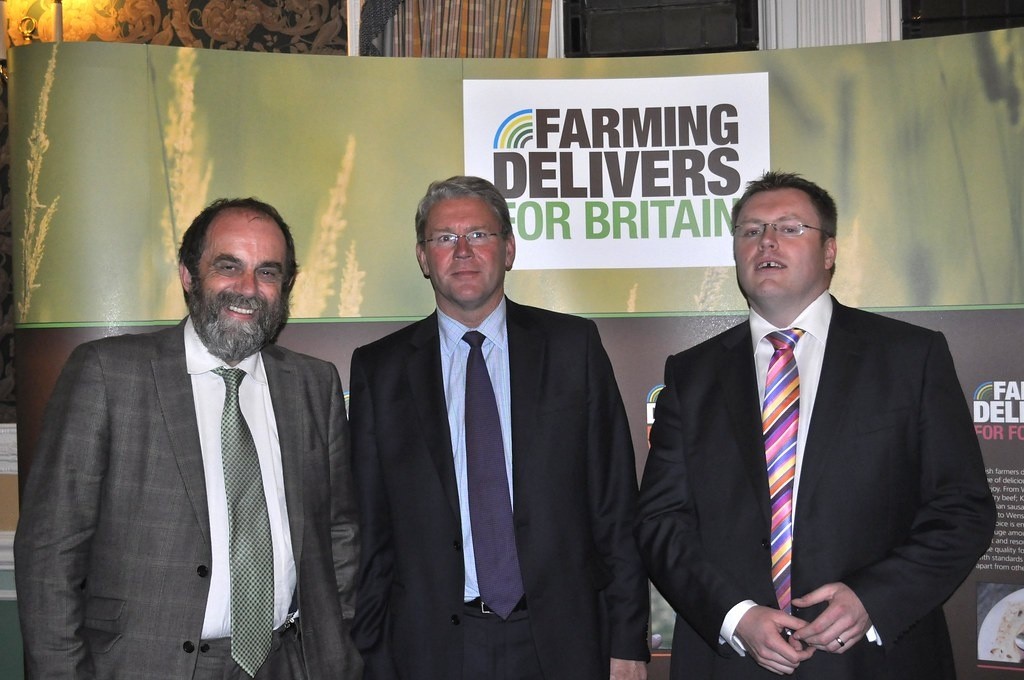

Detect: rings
835;637;844;647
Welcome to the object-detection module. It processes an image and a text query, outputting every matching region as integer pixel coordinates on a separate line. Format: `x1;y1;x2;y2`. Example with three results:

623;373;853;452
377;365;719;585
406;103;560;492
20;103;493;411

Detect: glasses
417;229;509;247
731;217;834;239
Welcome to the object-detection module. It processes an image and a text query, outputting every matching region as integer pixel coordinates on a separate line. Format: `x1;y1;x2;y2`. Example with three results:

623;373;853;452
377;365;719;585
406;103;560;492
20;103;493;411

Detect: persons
344;175;652;679
637;169;998;679
13;195;363;680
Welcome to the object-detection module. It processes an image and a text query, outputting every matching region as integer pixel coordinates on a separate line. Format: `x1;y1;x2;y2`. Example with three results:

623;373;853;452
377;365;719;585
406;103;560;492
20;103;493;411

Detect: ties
760;326;807;637
460;327;526;622
210;365;278;677
